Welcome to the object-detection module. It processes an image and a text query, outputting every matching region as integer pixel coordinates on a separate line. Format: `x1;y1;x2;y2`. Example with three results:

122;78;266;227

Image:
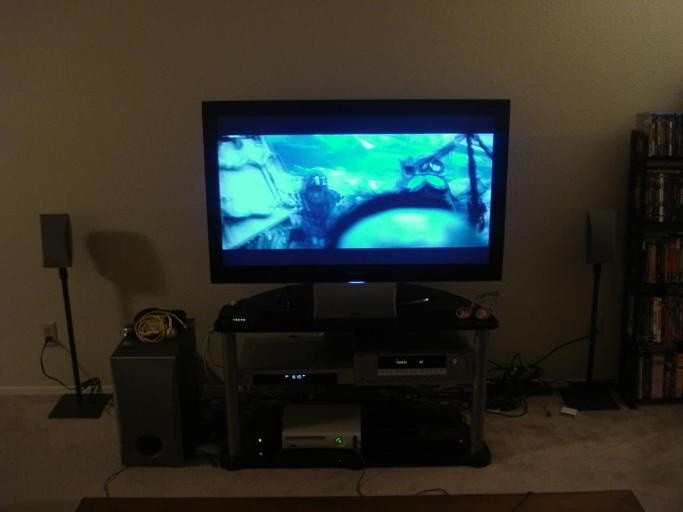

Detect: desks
76;489;648;512
214;281;499;471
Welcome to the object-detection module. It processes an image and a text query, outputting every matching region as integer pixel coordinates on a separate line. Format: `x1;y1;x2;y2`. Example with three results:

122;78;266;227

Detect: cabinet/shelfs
618;128;682;410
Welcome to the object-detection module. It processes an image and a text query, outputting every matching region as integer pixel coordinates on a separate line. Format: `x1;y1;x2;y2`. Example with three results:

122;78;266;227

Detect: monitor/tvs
201;97;512;318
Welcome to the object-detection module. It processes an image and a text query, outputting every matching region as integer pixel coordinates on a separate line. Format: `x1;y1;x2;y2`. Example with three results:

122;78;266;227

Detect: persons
295;170;342;239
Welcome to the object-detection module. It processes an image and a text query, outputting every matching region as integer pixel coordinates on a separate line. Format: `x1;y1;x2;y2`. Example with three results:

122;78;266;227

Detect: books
635;112;682;157
645;169;683;223
641;296;683;343
643;236;683;283
637;352;683;400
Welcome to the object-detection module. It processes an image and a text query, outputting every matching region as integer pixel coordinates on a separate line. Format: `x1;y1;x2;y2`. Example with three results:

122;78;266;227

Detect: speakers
40;212;73;270
584;209;615;264
108;317;199;467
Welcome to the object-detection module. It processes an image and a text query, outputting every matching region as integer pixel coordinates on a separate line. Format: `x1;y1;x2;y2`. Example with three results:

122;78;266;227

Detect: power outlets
41;321;57;347
